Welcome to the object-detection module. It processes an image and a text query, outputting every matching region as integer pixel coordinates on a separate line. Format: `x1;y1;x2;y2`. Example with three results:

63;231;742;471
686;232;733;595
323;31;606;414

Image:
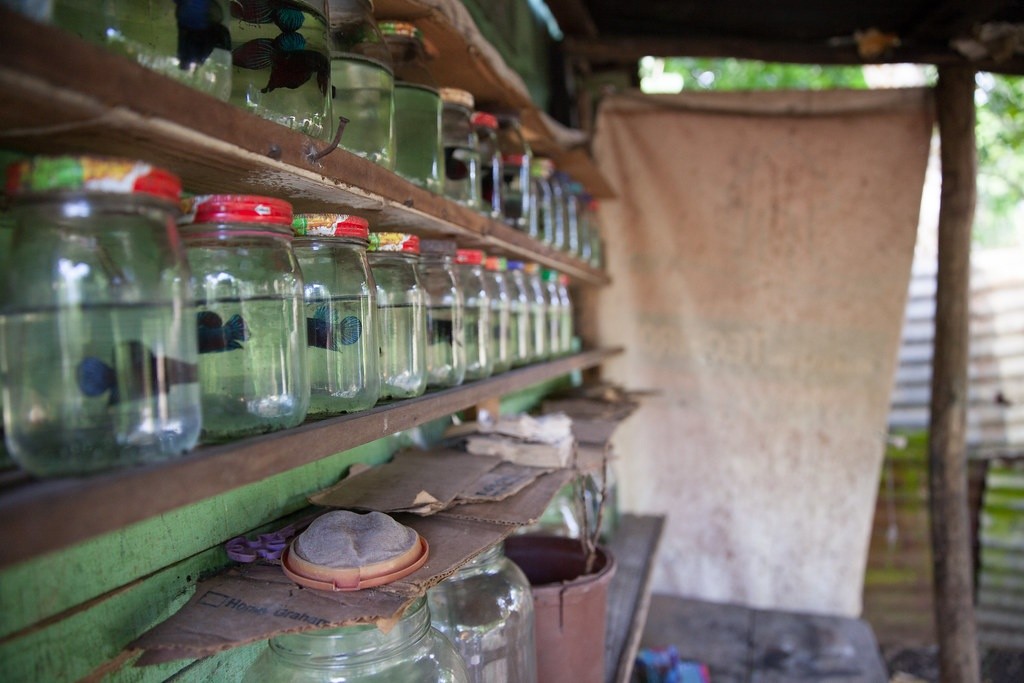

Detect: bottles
0;0;607;683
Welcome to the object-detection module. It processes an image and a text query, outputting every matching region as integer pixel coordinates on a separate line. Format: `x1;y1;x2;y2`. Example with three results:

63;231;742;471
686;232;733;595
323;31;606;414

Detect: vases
503;532;617;683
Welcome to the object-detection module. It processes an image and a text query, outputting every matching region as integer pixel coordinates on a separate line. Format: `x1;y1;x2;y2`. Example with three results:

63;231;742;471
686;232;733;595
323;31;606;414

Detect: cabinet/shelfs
0;0;671;683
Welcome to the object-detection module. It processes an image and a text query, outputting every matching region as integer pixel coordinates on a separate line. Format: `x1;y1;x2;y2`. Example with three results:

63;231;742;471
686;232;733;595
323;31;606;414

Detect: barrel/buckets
505;533;617;683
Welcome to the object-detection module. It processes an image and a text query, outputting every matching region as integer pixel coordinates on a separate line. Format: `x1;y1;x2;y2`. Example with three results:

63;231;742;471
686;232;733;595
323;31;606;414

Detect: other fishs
428;317;508;344
307;306;362;353
195;312;250;354
444;146;523;217
175;0;336;100
77;339;200;405
